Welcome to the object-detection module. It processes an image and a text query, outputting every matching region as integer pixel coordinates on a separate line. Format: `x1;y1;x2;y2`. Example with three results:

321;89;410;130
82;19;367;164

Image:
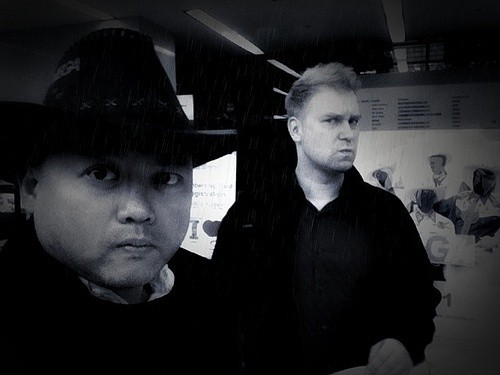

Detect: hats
407;178;445;204
465;160;500;173
427;143;447;160
371;154;396;178
0;27;237;184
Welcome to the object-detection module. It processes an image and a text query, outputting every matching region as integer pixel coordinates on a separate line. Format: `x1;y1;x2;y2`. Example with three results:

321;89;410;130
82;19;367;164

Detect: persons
447;164;500;256
0;74;273;374
373;167;410;211
210;59;446;375
418;151;467;206
408;180;457;271
